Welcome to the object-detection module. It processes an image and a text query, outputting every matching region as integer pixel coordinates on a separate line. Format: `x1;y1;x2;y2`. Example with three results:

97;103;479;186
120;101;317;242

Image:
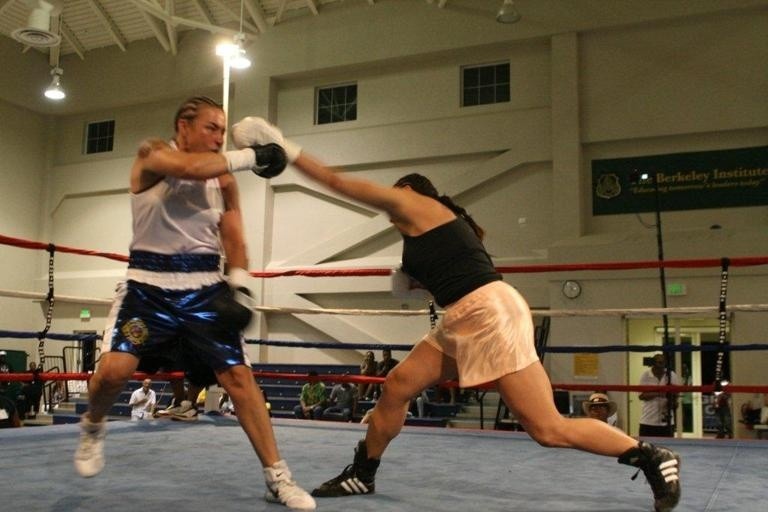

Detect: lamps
495;0;522;24
44;68;66;100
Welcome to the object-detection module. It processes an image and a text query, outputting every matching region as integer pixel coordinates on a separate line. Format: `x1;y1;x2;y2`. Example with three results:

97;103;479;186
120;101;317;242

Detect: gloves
250;143;287;178
391;268;419;296
217;287;252;331
231;114;285;150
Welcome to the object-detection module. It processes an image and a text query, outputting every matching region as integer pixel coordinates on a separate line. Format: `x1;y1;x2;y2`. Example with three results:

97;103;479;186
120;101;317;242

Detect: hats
583;393;618;415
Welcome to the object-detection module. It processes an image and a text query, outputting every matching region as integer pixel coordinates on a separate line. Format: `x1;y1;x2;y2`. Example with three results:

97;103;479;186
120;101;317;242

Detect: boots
74;413;108;478
309;439;380;497
156;397;199;421
617;438;682;511
263;459;317;511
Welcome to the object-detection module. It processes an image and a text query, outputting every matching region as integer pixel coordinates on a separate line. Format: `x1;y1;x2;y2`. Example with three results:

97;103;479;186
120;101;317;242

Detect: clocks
562;280;581;299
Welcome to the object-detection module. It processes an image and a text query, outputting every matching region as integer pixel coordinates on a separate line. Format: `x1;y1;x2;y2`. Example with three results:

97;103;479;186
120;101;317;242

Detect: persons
231;116;682;512
582;390;617;427
356;349;469;419
128;378;157;421
711;379;734;439
69;92;318;511
640;355;681;437
293;370;358;422
24;361;43;417
156;354;271;421
